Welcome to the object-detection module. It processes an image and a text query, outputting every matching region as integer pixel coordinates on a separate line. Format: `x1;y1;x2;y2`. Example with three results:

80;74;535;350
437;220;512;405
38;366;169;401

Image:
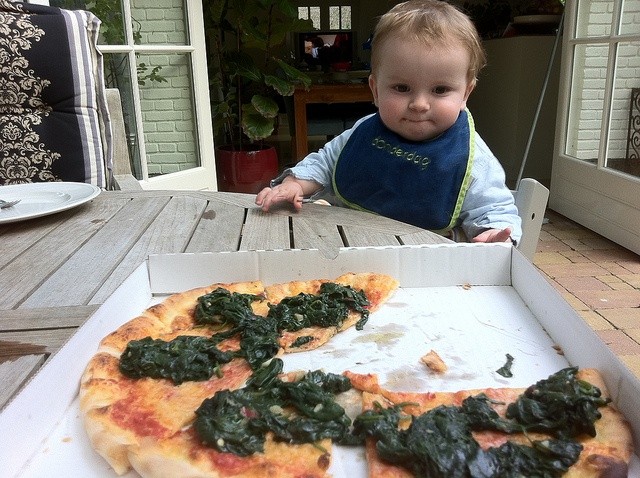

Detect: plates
0;181;101;224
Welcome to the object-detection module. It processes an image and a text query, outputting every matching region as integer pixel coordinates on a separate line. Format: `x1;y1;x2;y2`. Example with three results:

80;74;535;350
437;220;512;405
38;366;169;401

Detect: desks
293;81;374;163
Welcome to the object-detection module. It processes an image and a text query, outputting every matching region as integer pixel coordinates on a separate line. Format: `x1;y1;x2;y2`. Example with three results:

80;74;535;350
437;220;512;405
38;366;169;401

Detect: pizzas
79;272;400;477
343;368;635;477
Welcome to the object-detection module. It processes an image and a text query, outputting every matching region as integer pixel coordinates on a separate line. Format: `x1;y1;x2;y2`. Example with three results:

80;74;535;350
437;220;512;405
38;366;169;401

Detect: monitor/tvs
293;31;358;72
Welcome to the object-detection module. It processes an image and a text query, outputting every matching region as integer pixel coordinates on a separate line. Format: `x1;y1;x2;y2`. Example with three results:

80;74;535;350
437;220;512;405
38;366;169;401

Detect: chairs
103;88;143;190
509;177;551;264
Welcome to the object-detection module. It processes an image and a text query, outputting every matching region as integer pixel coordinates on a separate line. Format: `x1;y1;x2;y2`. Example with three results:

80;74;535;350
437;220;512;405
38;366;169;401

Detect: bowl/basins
331;63;352;72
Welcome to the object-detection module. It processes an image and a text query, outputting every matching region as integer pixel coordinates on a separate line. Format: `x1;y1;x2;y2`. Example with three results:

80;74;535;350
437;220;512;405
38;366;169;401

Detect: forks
0;199;21;209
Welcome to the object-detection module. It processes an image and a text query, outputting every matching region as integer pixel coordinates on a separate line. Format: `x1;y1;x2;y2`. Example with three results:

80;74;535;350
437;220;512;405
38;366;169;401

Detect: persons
255;0;523;244
304;35;350;64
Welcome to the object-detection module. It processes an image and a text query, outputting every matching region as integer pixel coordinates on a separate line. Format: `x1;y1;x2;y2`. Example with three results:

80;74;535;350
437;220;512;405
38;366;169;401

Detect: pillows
0;1;112;190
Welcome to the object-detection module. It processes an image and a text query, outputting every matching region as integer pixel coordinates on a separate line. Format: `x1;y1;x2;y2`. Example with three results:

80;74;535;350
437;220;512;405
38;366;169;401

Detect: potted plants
202;0;319;193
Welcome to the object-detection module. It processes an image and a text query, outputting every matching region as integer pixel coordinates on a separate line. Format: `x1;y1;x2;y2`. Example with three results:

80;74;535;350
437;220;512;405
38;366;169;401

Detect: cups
316;65;322;72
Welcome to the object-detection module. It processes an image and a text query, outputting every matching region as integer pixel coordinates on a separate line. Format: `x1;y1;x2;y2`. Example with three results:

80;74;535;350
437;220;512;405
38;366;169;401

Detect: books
330;62;372;84
